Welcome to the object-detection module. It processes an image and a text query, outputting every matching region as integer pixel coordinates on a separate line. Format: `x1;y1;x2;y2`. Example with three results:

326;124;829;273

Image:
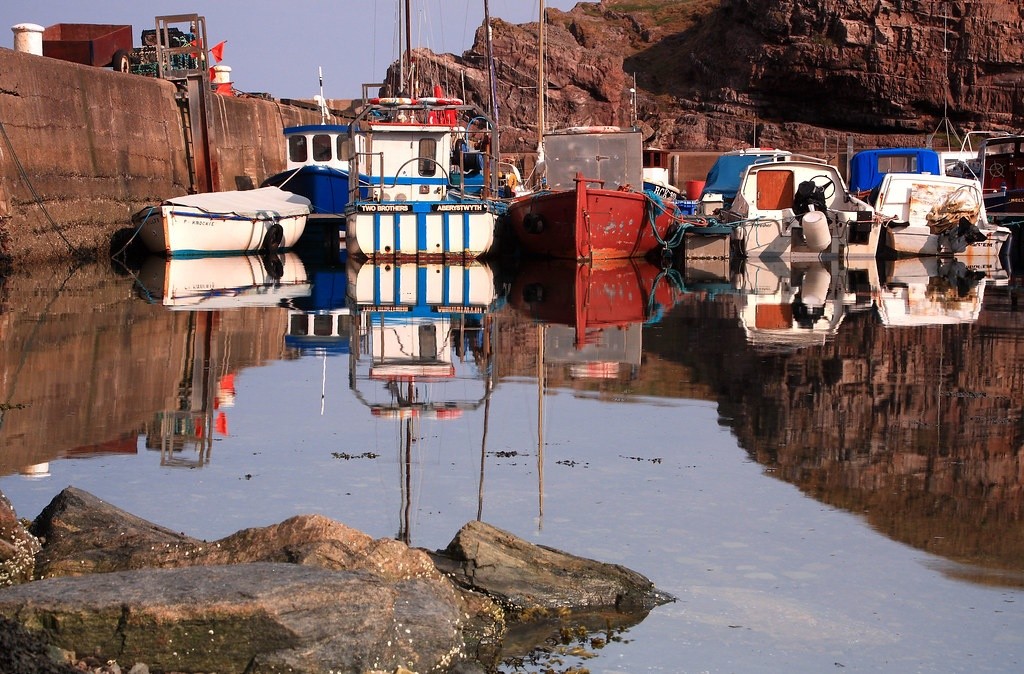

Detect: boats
344;0;494;263
129;184;312;257
131;250;313;311
491;258;687;381
283;236;348;417
258;65;371;237
495;0;1024;261
679;259;854;350
345;255;499;551
844;254;1011;328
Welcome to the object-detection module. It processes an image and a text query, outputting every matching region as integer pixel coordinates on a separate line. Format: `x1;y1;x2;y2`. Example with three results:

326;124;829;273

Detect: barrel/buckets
802;263;831;306
685;180;705;200
802;211;832;249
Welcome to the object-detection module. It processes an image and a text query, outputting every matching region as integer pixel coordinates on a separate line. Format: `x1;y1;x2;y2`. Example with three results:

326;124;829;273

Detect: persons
504;172;516;198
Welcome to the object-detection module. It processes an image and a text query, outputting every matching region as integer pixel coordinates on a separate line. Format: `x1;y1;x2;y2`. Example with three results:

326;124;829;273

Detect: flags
211;42;224;62
195;418;203;439
208;67;216;82
189;38;203;59
219;373;235;390
216;84;231;95
215;413;227;436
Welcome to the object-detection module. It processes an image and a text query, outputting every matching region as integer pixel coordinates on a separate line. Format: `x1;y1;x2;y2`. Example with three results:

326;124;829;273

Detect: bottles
1001;180;1007;192
